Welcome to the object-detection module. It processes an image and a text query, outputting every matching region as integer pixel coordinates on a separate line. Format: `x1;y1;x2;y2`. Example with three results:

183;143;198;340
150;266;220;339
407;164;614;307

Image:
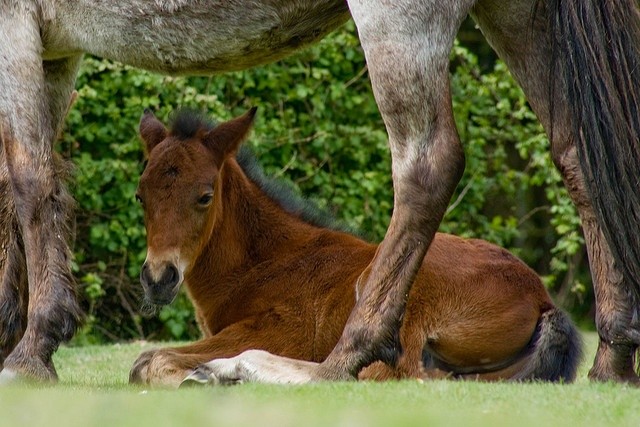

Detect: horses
0;0;640;386
129;105;585;389
